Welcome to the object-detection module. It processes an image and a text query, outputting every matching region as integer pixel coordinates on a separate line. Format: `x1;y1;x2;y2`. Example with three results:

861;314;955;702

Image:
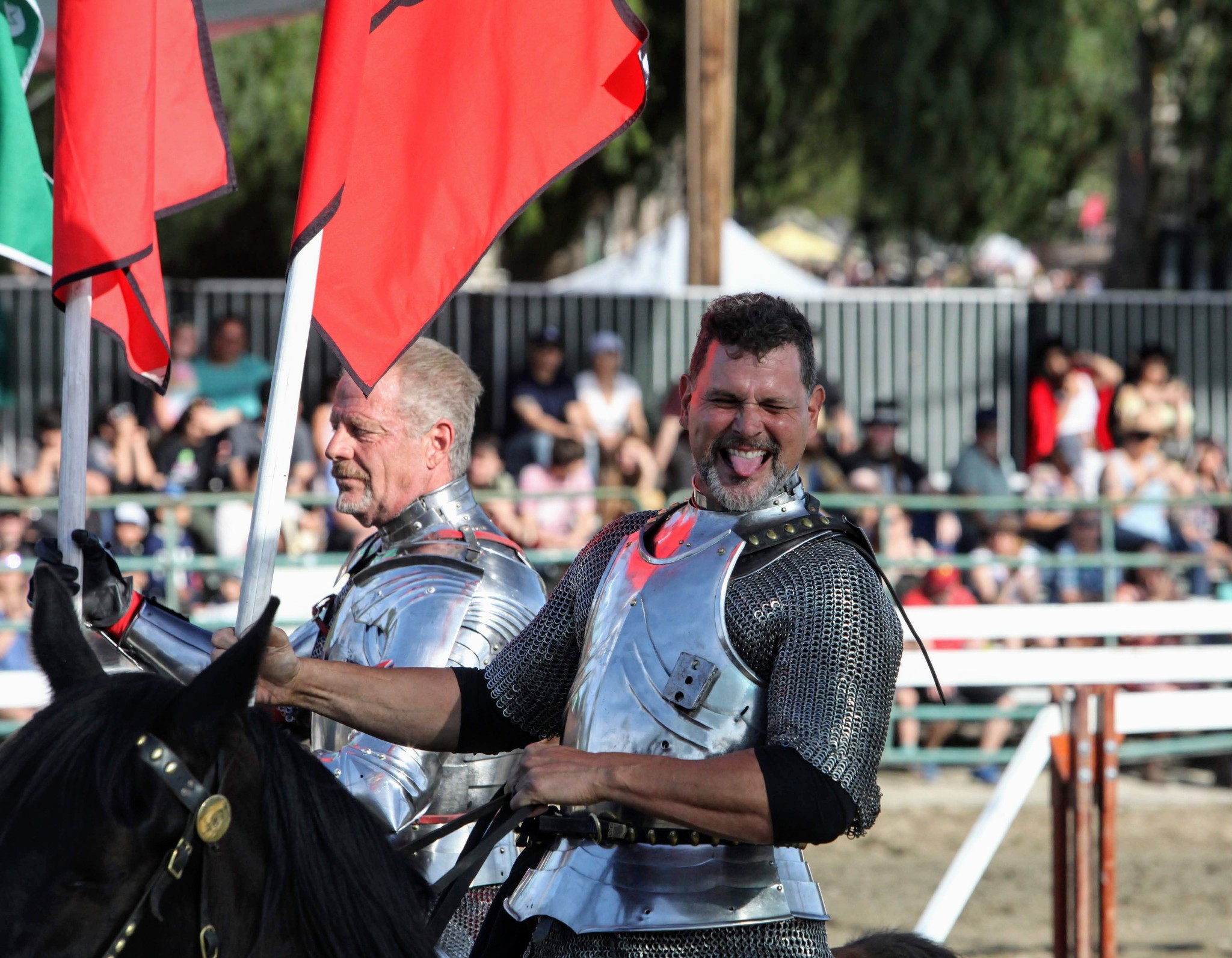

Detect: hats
114;502;152;534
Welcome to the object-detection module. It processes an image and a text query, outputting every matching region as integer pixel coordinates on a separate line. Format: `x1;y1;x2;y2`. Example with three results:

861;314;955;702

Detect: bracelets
103;589;144;641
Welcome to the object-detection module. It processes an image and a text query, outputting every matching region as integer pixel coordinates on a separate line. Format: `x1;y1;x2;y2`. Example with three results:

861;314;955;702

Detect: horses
0;561;962;958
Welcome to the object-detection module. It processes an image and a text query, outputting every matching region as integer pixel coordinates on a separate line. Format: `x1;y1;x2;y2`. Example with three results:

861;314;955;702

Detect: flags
49;0;240;396
284;0;653;398
0;0;56;269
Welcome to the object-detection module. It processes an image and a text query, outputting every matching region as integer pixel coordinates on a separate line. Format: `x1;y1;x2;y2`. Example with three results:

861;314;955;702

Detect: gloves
28;529;136;630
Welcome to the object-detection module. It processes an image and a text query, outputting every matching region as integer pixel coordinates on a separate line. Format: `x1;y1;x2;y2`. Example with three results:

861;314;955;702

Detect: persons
24;337;550;958
208;293;948;958
0;237;1230;790
